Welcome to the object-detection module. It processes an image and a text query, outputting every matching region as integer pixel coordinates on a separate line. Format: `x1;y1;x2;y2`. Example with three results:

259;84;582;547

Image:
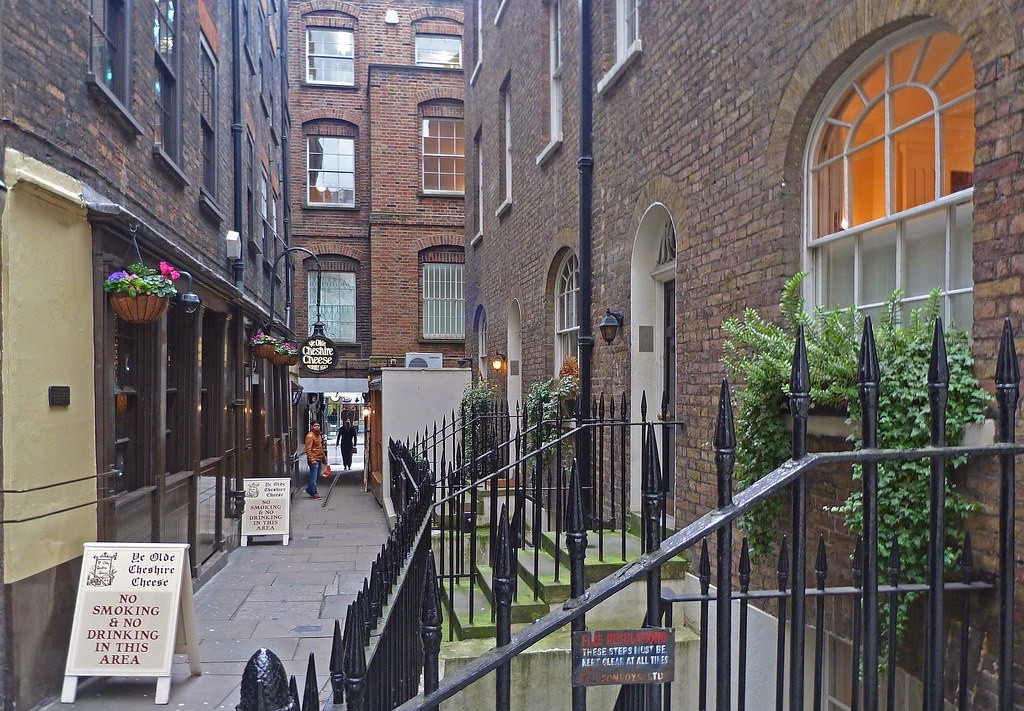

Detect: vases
290;356;298;366
252;343;275;359
269;354;290;365
110;292;169;323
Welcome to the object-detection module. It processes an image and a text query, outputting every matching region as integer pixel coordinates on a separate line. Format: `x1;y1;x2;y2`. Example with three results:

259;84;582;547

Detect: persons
337;422;357;470
305;420;327;499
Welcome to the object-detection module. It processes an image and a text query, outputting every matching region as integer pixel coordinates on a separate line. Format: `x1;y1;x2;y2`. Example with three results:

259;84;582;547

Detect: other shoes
312;493;322;501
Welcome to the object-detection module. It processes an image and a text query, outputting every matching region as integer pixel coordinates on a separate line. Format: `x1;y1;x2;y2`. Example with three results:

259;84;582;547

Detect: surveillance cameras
180;294;200;314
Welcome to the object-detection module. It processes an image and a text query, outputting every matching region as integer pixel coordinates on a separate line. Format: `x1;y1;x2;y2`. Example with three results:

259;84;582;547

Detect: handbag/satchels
318;462;332;481
351;445;357;453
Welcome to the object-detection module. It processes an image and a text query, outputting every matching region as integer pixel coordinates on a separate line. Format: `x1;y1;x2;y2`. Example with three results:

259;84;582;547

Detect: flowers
287;345;300;357
273;340;289;356
101;259;180;299
248;333;277;347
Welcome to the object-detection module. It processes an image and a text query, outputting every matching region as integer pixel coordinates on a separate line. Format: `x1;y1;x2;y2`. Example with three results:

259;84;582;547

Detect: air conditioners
405;351;443;368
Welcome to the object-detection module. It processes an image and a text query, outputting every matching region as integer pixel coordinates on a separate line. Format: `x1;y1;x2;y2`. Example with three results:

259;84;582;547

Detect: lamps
491;349;506;372
598;307;624;346
177;271;201;314
363;404;371;417
291;380;303;406
355;394;362;403
